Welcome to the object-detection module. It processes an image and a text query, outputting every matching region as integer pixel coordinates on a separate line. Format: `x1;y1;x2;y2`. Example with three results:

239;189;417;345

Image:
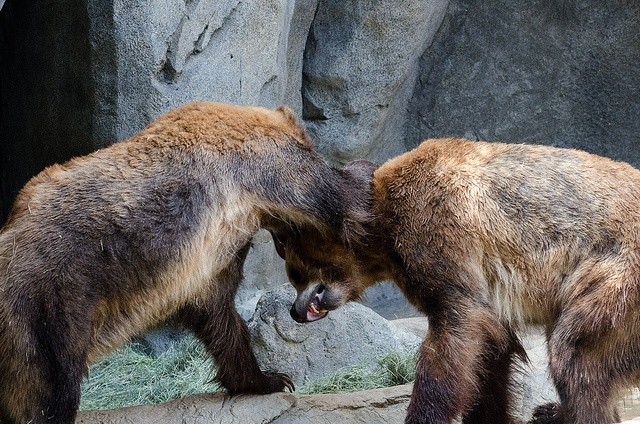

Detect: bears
285;135;640;424
0;99;382;424
